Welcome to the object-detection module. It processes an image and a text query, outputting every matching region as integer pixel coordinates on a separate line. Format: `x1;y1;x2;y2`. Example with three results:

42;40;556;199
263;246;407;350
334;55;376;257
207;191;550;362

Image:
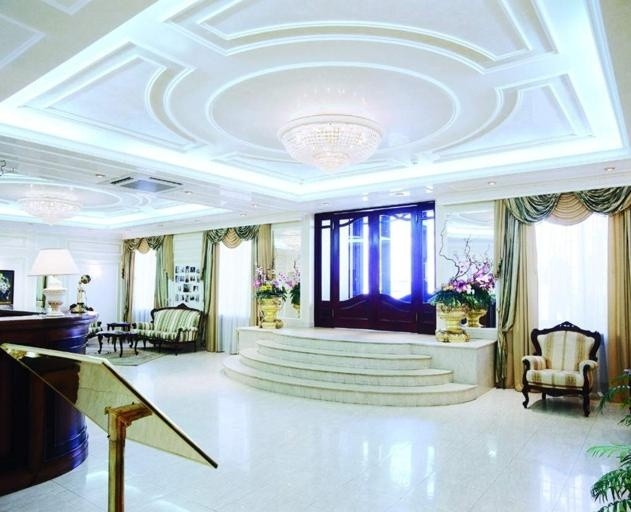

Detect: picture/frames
0;269;15;305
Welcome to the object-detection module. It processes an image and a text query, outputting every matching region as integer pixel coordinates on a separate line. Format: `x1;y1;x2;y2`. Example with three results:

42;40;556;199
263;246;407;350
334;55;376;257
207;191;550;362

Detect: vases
256;296;284;329
466;305;488;327
437;303;471;343
293;304;300;318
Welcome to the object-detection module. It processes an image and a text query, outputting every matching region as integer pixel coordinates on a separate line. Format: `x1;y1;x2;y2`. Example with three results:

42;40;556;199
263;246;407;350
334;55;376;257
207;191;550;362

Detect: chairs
521;321;602;416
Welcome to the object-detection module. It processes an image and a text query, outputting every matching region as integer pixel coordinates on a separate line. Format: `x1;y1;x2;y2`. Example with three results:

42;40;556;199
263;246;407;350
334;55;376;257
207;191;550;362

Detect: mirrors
438;204;497;328
272;222;300;317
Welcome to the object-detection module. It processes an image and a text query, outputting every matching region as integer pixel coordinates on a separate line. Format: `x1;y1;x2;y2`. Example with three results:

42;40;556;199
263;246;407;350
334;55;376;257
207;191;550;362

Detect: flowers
429;220;475;309
286;256;301;304
468;255;497;309
255;255;289;303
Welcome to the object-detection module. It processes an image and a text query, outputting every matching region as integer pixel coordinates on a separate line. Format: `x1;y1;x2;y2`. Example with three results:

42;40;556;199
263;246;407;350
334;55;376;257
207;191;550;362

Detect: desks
96;330;140;357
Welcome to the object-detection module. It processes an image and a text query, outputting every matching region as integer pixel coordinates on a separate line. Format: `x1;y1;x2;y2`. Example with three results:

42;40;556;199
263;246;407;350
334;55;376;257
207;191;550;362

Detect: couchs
133;302;203;356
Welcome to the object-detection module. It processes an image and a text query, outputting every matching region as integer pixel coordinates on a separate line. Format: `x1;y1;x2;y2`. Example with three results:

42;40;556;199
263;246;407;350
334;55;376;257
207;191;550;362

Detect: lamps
281;112;386;175
27;249;80;317
